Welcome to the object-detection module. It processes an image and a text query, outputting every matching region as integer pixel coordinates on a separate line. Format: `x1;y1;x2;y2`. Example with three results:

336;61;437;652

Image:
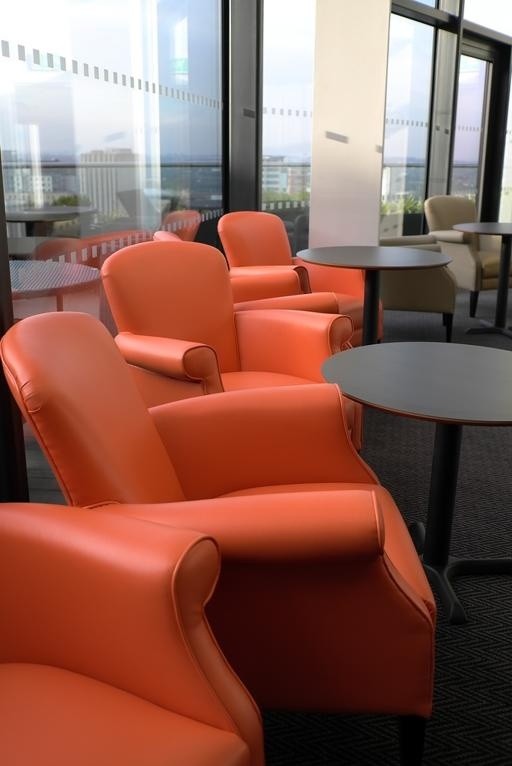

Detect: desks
8;237;64;260
9;259;101;312
321;343;512;623
297;247;451;346
6;211;77;237
452;222;512;338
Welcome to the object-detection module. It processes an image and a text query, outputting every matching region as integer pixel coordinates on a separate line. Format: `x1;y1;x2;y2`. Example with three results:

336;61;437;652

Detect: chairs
425;196;512;318
37;211;202;333
101;241;362;457
218;211;384;346
380;234;457;342
153;230;353;353
0;502;267;766
0;312;435;766
